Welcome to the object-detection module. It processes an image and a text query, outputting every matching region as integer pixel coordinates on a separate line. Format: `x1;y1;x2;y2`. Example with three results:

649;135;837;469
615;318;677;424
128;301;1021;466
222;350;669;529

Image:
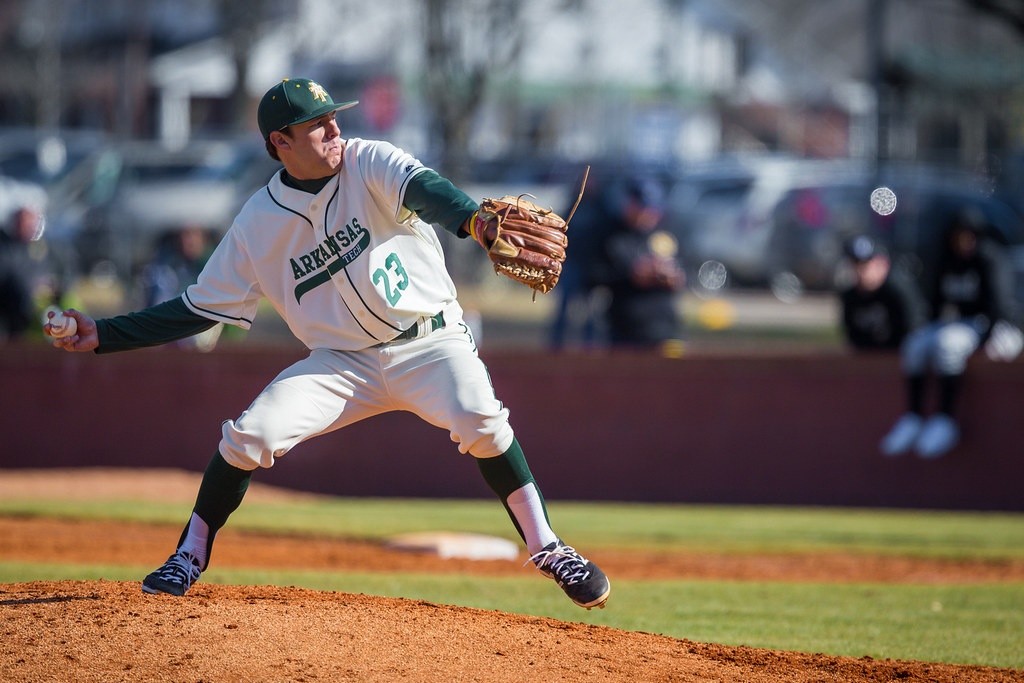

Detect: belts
391;311;445;340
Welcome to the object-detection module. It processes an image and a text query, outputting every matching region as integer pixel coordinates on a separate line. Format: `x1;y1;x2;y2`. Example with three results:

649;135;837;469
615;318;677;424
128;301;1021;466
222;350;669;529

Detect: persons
880;222;1023;458
44;78;611;612
550;156;689;348
839;234;918;352
0;199;55;337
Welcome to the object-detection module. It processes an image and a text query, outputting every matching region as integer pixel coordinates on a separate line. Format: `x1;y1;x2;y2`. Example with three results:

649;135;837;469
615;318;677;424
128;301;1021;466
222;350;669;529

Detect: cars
669;156;1015;291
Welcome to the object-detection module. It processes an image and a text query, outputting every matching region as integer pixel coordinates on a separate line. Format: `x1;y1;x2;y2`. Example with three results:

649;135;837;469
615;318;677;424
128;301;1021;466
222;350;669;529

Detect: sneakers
523;537;610;611
142;548;202;597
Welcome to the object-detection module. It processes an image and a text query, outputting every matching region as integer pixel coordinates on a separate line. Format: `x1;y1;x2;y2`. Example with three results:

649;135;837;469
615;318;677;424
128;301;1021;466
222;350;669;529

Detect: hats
258;78;360;140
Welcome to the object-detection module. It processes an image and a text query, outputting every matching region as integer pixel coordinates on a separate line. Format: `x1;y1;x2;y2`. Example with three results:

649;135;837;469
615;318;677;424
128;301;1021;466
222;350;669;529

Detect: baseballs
48;311;78;339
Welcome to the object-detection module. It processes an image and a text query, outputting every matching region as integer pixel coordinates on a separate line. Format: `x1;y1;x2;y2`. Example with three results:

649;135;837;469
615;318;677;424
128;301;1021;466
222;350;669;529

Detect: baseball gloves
469;192;569;295
